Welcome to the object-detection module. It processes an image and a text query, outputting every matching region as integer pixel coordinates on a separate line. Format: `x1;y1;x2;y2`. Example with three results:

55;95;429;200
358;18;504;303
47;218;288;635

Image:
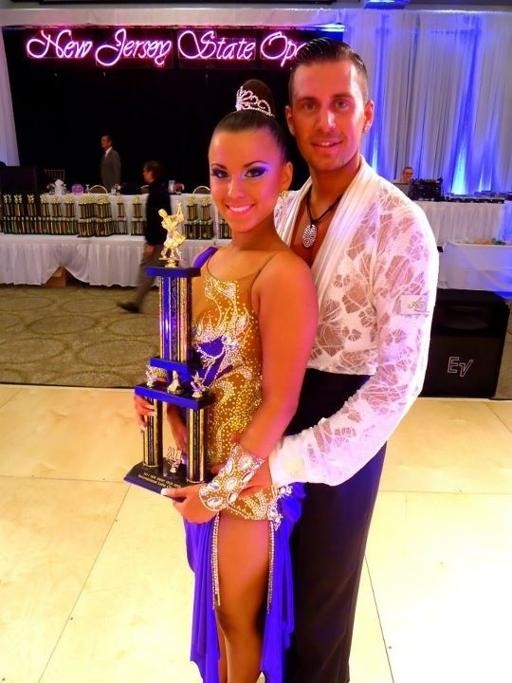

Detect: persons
100;135;121;193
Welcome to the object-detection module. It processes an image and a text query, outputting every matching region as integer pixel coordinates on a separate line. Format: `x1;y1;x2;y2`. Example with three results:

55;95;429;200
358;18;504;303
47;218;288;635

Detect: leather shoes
118;301;141;313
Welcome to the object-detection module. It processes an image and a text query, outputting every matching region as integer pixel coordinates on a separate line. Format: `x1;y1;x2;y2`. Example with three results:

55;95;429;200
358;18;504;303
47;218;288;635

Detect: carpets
0;284;162;387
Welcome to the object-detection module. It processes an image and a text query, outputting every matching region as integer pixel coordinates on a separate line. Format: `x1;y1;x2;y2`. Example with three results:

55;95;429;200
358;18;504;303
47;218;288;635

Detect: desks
0;188;294;289
411;198;510;290
